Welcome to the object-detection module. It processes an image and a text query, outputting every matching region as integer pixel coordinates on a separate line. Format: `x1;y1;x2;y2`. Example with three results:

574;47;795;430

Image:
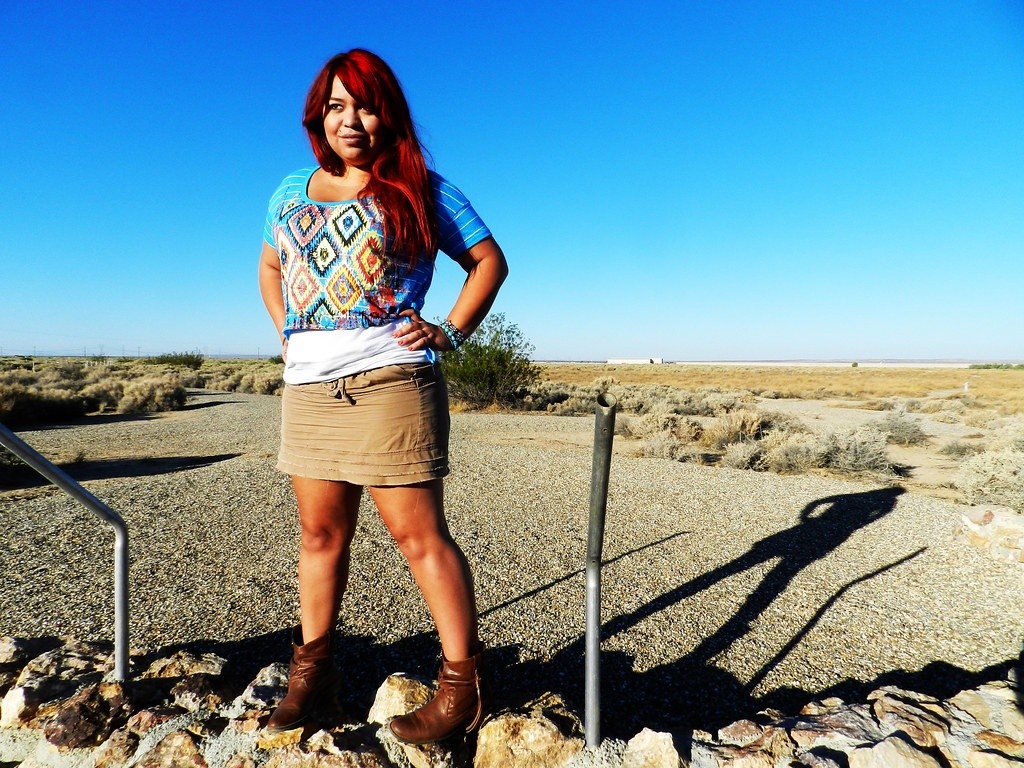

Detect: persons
259;50;508;744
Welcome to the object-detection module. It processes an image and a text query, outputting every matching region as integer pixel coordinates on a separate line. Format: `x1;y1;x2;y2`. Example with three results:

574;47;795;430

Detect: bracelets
434;316;465;349
283;337;286;345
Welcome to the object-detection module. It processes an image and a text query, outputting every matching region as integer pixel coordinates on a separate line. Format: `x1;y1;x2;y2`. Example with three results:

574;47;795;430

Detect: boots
391;642;486;744
267;624;336;732
